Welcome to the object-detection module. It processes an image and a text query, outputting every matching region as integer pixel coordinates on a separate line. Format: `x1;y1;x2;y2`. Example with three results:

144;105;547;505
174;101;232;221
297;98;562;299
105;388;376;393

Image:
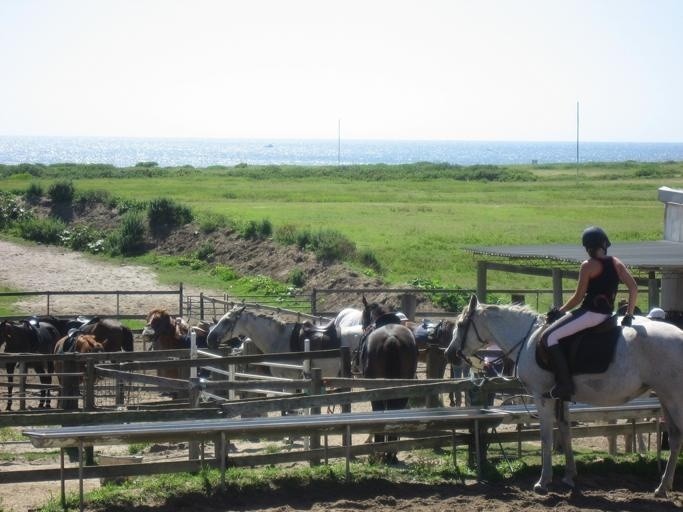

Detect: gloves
621;313;634;326
546;308;566;322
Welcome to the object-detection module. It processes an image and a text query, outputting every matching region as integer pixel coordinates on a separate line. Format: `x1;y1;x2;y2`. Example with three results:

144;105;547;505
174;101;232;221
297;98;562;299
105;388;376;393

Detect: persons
173;315;210;348
645;308;666;322
542;225;638;399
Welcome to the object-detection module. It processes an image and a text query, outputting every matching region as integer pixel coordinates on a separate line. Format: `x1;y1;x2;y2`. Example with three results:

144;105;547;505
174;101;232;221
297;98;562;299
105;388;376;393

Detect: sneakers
543;375;574;398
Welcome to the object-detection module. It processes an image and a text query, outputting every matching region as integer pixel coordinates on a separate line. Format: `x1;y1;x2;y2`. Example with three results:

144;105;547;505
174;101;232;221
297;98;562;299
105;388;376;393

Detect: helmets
581;227;613;248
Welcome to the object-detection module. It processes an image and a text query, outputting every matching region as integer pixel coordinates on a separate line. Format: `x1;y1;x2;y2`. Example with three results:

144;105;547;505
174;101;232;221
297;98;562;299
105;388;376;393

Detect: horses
0;315;133;410
141;309;188;399
206;303;376;444
328;293;683;499
